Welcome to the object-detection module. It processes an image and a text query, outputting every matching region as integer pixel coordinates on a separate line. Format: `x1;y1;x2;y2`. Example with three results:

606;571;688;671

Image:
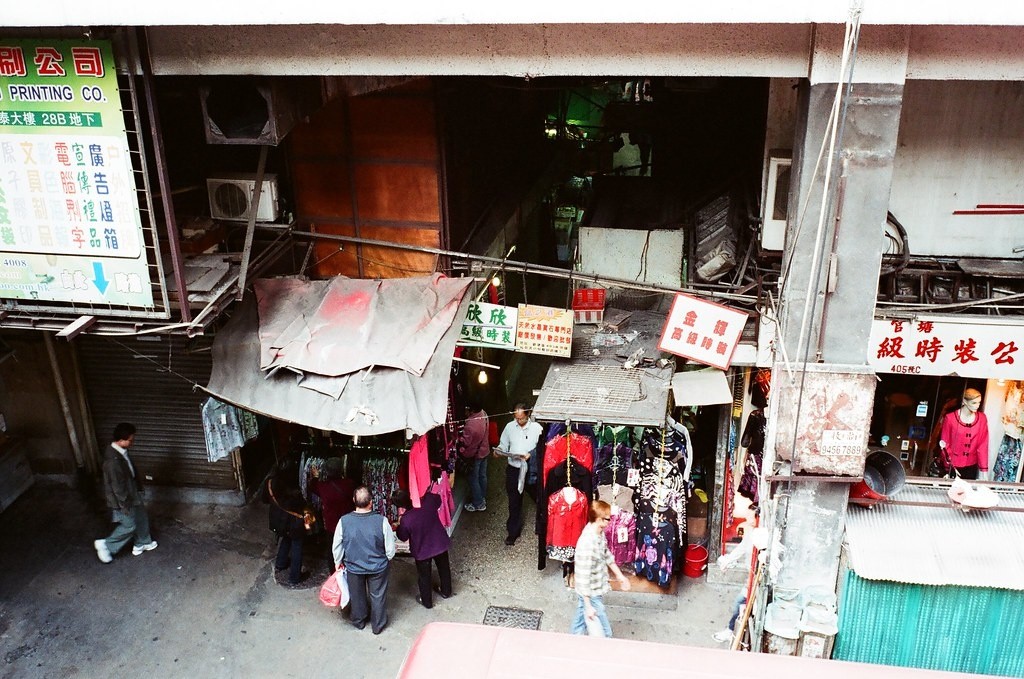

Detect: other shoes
505;532;520;544
433;584;449;598
712;630;732;642
288;569;312;584
416;594;433;608
275;557;293;571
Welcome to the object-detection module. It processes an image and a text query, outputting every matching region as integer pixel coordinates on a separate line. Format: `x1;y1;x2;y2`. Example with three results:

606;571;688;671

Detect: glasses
603;518;611;522
516;415;528;421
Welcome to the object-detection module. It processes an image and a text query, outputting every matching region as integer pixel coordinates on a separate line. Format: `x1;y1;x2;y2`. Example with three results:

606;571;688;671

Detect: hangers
435;471;443;484
303;443;405;468
557;412;678;516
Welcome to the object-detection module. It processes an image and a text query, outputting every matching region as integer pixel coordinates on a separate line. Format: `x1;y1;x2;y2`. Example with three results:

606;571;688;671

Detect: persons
460;399;489;511
263;459;311;583
333;486;396;634
393;489;452;608
94;423;158;563
310;457;356;575
712;502;760;642
939;388;989;481
572;500;631;638
494;403;543;545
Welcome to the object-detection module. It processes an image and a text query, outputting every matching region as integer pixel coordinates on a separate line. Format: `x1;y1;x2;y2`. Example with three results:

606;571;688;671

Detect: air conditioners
207;173;282;221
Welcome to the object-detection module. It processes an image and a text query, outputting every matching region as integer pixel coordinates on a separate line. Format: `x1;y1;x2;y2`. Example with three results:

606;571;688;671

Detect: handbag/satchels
319;566;344;607
455;456;473;474
303;508;319;535
336;570;351;609
928;448;951;478
583;607;605;636
517;461;528;494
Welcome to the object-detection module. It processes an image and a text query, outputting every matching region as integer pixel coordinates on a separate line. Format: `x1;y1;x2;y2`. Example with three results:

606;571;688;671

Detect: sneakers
464;503;486;511
94;539;113;563
132;540;158;555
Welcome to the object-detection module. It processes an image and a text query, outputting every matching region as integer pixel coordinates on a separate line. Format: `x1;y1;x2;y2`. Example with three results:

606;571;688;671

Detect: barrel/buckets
682;544;709;578
852;450;905;508
